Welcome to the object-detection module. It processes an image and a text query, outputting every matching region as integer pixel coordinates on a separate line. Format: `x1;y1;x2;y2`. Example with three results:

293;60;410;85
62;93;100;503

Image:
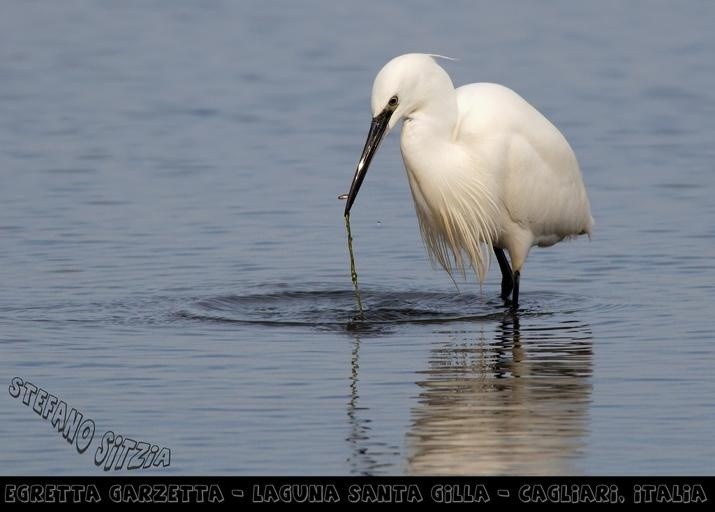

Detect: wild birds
344;52;595;308
